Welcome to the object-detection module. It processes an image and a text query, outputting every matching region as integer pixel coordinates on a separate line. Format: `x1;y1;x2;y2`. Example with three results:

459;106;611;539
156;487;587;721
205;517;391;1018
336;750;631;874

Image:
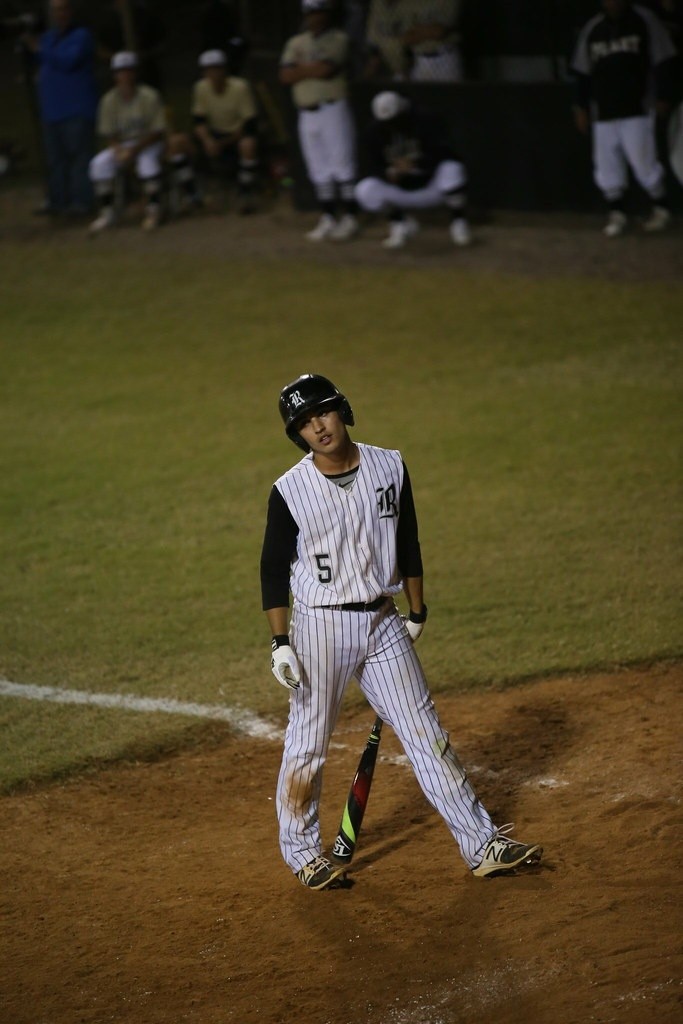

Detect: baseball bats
329;713;384;861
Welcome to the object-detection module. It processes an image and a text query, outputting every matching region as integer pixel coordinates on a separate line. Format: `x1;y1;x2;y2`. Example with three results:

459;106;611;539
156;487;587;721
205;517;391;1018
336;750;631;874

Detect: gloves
270;635;302;690
405;603;428;640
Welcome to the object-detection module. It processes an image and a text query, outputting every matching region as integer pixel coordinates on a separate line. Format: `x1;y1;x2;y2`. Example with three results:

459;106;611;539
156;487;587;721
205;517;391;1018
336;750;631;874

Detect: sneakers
295;856;347;890
471;823;543;877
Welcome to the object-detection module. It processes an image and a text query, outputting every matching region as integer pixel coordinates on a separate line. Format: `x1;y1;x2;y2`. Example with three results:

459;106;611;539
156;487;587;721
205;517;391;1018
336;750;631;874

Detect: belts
321;596;389;612
304;100;335;111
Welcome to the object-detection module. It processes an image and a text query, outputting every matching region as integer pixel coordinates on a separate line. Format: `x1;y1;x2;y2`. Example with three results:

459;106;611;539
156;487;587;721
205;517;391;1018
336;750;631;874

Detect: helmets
279;373;355;454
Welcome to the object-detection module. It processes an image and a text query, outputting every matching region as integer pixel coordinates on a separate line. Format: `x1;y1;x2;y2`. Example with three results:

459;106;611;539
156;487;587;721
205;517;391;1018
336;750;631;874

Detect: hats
199;49;226;66
372;91;409;121
304;0;321;12
112;50;136;68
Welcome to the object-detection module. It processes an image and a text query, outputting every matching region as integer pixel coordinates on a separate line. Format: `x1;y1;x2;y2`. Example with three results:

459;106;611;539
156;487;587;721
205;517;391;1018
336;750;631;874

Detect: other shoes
643;205;671;230
307;214;334;239
603;210;626;234
382;215;418;248
89;211;118;229
450;219;471;245
333;214;359;238
141;210;163;229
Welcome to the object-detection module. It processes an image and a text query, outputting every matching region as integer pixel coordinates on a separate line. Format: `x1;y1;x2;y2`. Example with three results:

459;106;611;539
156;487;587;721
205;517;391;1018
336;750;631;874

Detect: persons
560;0;683;238
22;0;475;246
260;374;543;890
